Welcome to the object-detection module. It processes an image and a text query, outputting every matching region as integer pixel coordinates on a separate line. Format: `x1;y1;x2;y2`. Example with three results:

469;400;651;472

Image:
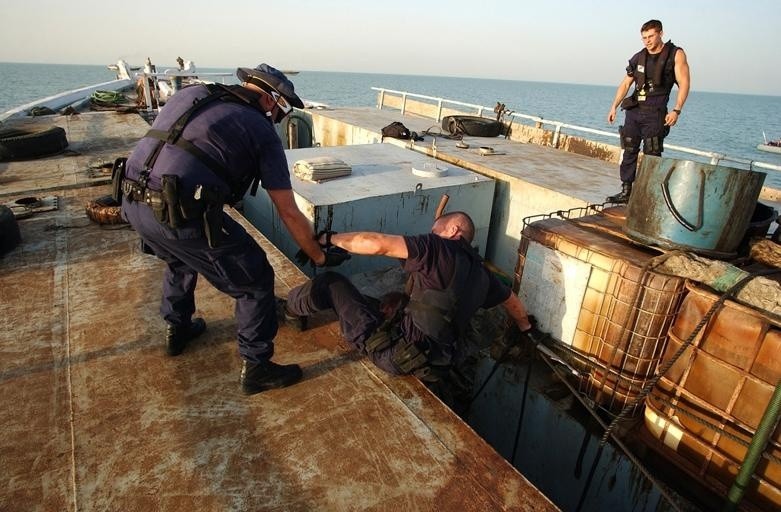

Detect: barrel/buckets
622;155;768;260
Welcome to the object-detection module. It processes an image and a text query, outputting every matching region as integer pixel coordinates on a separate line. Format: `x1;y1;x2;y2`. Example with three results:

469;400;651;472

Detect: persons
176;56;185;70
606;19;690;204
272;211;540;383
112;63;353;395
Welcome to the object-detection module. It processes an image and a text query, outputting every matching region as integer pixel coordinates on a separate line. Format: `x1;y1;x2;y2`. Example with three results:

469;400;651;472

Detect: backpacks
380;122;424;144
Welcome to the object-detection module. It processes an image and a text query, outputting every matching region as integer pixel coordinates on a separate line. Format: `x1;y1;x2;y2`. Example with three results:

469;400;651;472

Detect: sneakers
272;296;308;333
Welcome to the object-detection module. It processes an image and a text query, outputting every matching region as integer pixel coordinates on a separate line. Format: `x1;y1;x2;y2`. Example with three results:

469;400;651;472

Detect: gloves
310;246;351;268
294;231;337;266
524;328;556;355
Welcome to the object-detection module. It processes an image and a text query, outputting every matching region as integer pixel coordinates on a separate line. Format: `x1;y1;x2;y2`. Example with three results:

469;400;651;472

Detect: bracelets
326;230;337;246
673;109;682;114
310;249;329;269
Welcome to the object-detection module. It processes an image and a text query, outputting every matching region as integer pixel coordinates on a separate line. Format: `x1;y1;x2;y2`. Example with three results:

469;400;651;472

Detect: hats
237;63;304;110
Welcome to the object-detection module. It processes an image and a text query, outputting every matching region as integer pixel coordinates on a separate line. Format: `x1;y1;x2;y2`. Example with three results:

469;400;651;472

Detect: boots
240;359;303;396
606;182;630;203
165;318;205;357
